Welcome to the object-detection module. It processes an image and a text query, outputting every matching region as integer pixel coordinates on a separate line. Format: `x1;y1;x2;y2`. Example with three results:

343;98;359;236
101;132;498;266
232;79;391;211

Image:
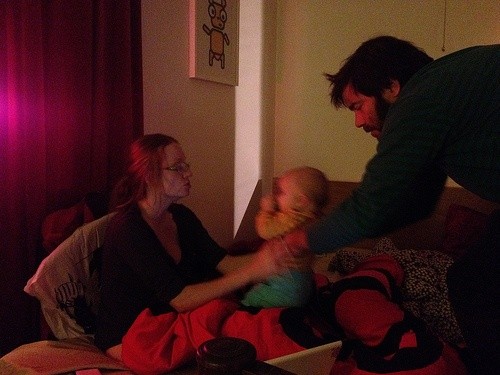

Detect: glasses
161;160;192;172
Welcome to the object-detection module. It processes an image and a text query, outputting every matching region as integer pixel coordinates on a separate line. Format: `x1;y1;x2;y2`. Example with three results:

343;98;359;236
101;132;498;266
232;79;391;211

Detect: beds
0;179;500;375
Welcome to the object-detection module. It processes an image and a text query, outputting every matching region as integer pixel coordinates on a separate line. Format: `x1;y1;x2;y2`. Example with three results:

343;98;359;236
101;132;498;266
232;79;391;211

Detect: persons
239;166;333;308
94;134;307;362
279;34;499;374
